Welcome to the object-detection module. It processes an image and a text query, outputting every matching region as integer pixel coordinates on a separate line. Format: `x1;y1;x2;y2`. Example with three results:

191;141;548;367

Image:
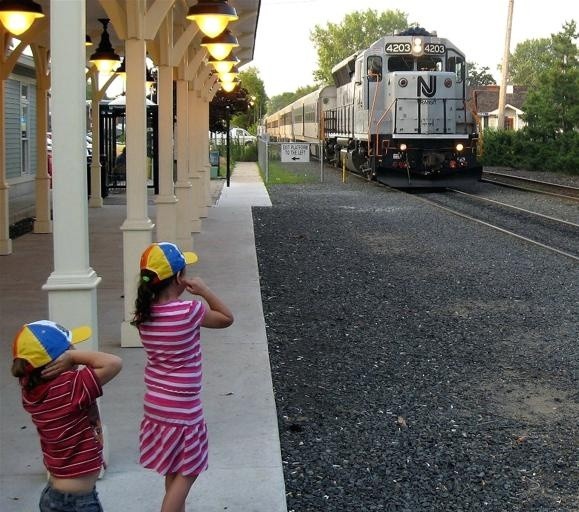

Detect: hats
140;242;199;285
12;319;92;369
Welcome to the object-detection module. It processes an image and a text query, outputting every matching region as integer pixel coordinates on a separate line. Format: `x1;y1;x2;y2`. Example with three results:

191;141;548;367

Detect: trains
262;27;483;181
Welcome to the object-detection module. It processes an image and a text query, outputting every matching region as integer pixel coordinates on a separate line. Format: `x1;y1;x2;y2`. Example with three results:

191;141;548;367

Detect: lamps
0;0;45;35
86;17;125;75
146;64;157;88
187;0;241;93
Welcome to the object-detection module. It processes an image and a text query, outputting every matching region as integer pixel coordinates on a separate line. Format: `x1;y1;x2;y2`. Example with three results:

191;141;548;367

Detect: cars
212;128;257;146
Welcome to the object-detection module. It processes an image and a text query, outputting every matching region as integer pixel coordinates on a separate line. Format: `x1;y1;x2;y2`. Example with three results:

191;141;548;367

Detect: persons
112;147;126;187
131;242;234;512
11;319;124;512
367;63;380;82
